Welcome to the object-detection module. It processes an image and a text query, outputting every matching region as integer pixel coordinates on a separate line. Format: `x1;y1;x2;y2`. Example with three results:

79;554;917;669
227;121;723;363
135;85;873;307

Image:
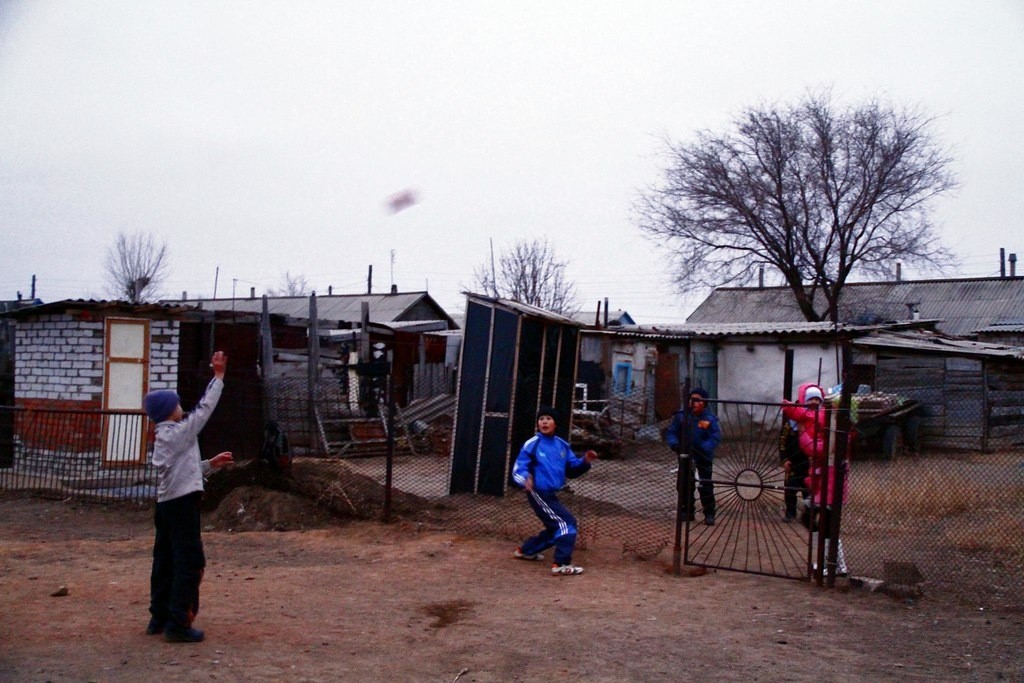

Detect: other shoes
680;512;695;521
162;624;203;643
146;619;163;634
782;516;794;522
804;499;811;509
705;514;714;525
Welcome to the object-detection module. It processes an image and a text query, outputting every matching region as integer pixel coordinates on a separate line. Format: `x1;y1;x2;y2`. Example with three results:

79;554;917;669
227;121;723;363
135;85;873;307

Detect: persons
143;352;235;643
780;383;858;576
666;388;722;525
780;400;813;523
512;407;597;576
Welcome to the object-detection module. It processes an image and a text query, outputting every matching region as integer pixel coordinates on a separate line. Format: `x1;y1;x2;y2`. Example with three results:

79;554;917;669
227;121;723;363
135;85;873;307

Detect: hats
143;389;180;423
690;388;708;408
804;386;824;404
536;406;560;425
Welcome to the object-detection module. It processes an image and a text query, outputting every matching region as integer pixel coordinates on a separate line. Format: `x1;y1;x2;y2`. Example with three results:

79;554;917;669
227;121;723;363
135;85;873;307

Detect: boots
812;537;846;576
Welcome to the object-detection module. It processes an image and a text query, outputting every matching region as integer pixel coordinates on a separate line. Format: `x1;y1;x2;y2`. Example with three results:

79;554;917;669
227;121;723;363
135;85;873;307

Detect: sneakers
514;547;544;560
551;563;584;576
